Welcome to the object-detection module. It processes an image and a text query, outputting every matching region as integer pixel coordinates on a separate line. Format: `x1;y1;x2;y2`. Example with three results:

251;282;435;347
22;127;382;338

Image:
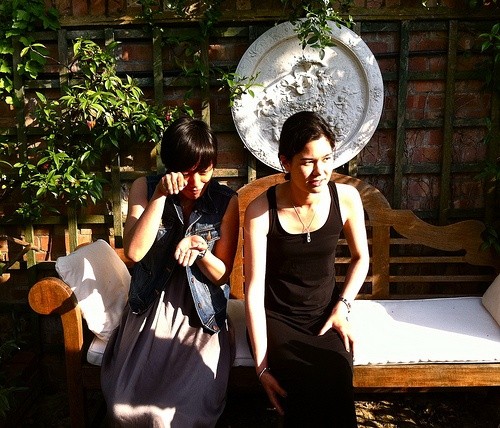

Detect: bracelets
338;296;351;314
258;367;271;380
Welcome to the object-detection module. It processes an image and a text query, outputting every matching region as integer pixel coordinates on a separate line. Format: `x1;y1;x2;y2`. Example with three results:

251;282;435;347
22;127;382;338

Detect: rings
273;407;277;412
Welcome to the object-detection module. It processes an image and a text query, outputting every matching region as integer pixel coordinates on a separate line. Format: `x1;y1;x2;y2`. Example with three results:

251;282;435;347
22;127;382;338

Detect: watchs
195;248;207;260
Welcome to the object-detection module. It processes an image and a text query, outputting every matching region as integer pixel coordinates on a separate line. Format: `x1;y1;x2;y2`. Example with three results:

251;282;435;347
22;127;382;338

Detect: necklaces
288;193;323;243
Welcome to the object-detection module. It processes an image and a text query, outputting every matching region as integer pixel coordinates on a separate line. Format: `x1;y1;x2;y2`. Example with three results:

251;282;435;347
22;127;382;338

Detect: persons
102;116;240;428
244;110;371;428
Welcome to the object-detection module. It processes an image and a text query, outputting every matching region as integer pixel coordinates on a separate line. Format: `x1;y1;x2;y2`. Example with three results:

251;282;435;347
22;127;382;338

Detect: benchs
29;173;500;428
